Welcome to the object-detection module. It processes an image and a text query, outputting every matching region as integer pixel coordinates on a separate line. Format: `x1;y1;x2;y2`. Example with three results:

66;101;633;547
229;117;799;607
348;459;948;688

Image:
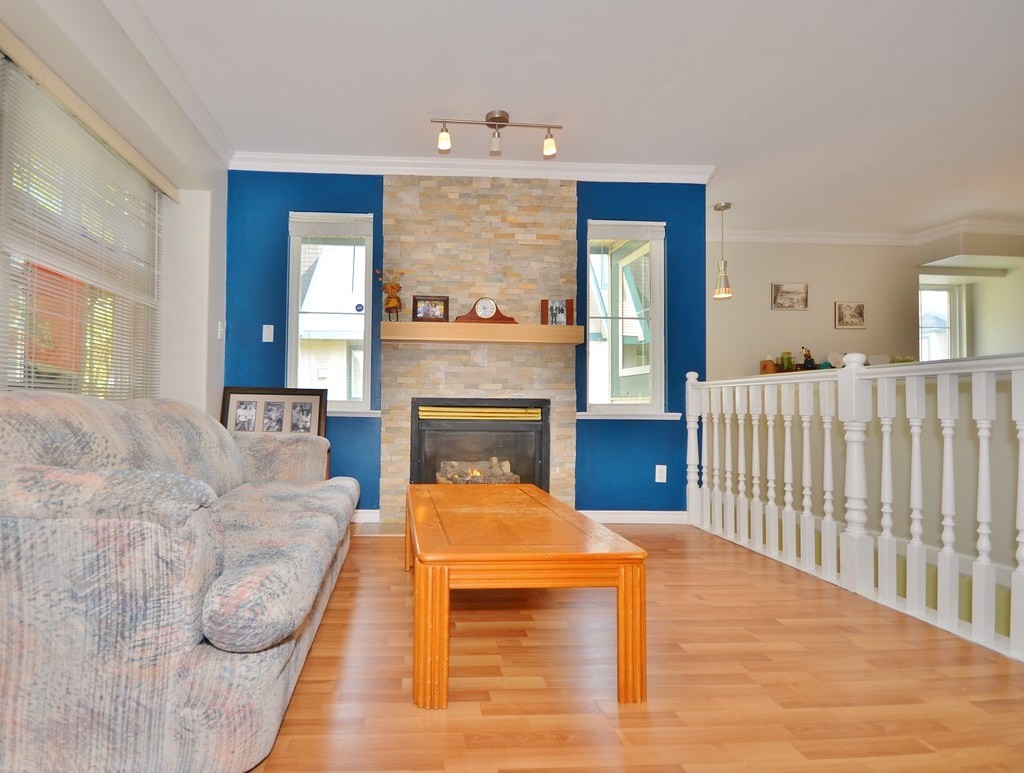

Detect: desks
759;360;820;374
405;483;648;710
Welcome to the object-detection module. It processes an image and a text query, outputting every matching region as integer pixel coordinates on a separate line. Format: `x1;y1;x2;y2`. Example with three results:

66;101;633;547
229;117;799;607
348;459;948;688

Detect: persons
549;302;565;325
422;301;440;318
235;402;311;432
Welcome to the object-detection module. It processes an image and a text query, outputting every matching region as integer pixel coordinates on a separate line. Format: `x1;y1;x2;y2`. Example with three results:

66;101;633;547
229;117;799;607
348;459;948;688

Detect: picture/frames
835;302;867;329
220;387;328;438
541;298;574;325
412;294;449;322
771;281;810;312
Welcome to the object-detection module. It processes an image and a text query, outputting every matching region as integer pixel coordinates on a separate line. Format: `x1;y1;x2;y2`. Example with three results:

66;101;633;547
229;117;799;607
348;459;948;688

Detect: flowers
376;269;405;285
800;346;813;359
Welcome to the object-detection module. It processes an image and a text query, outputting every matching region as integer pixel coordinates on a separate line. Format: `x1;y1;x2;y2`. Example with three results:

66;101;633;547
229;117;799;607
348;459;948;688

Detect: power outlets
655;464;667;483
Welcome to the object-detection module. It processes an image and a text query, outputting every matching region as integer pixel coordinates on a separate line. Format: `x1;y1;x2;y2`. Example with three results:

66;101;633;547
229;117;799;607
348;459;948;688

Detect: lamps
431;110;563;156
712;203;733;298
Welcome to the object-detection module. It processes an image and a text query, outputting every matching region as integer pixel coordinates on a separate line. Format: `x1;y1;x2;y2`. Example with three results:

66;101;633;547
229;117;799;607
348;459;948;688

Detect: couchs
0;391;360;773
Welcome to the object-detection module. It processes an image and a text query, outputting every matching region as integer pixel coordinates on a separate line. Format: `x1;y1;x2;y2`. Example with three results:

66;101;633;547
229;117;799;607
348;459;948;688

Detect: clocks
453;296;521;324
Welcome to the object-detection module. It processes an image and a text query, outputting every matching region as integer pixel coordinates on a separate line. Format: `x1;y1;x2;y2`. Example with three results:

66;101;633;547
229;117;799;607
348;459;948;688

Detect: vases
382;285;403;321
803;359;816;369
